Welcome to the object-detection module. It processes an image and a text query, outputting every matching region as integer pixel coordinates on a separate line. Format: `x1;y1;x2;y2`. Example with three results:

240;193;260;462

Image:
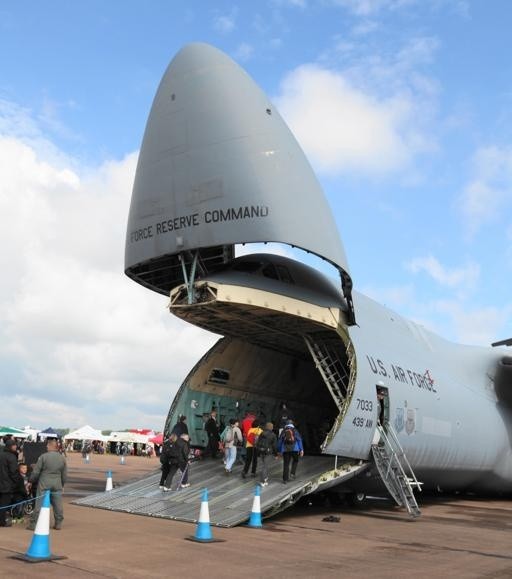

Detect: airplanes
57;40;512;536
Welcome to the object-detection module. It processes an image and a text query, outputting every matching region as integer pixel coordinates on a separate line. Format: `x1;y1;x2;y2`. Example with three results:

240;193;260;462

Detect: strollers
8;482;36;516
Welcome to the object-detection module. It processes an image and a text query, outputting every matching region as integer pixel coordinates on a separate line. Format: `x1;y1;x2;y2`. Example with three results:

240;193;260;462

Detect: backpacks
284;428;296;445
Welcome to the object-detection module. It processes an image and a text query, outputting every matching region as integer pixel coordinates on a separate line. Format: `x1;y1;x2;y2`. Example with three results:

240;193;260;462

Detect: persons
202;411;303;486
377;388;385;424
66;439;154;459
1;434;67;530
120;446;127;465
81;443;91;464
160;416;192;490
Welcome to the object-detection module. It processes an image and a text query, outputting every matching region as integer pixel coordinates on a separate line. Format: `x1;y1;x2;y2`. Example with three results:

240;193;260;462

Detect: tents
61;424;163;454
1;424;62;439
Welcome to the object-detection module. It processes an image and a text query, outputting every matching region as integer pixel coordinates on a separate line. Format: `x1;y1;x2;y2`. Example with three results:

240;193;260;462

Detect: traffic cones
10;490;70;563
243;484;269;528
185;487;228;544
84;454;91;464
105;470;115;493
118;454;126;465
